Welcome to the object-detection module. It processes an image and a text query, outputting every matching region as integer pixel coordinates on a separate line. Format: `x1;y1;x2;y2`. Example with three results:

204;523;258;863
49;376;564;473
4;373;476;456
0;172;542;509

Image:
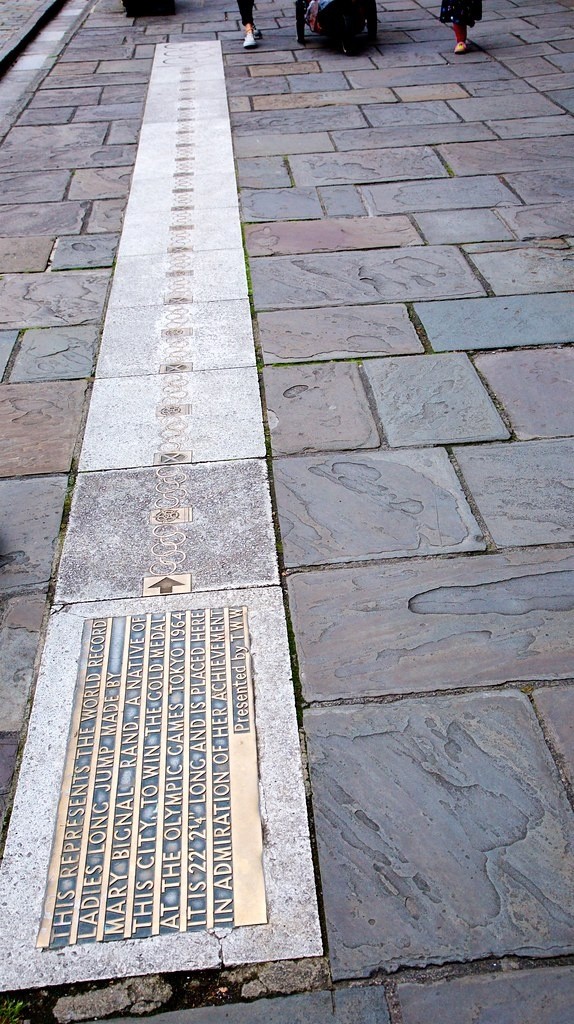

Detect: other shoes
244;36;257;48
454;41;467;54
251;25;260;38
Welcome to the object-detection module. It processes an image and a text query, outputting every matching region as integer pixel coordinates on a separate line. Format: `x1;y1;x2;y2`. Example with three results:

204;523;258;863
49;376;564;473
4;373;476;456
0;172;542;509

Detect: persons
237;0;262;48
439;0;482;54
